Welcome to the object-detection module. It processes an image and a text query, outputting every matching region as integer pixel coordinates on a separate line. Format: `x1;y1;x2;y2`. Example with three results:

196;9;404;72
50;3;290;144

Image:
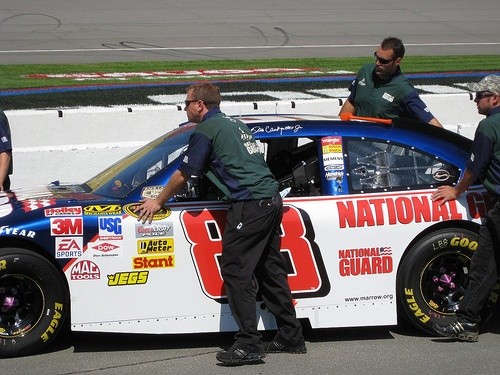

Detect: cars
0;112;500;356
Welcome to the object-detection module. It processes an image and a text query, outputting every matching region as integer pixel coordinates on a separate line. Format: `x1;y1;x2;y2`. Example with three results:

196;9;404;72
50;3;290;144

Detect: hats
465;75;500;94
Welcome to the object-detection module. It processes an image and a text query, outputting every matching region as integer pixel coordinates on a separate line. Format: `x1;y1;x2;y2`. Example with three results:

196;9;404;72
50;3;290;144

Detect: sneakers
215;345;267;364
264;336;309;355
432;317;480;342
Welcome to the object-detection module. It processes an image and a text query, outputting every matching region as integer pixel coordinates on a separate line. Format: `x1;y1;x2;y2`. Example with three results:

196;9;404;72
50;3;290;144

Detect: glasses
373;51;396;64
185;100;197;105
477;91;494;98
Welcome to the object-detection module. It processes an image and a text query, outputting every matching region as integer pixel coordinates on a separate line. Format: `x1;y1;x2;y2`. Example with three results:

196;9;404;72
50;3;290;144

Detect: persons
338;36;445;130
0;108;14;191
430;73;500;342
135;82;308;364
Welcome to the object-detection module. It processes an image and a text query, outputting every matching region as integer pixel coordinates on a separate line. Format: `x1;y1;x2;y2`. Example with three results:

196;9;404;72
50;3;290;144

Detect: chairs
268;149;298;180
